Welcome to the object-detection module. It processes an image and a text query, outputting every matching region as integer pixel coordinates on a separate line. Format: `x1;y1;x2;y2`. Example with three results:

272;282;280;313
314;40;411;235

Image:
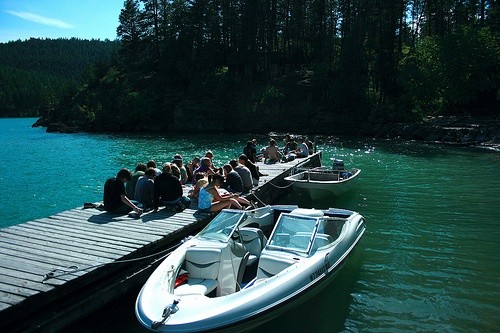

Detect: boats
284;167;362;203
135;204;367;333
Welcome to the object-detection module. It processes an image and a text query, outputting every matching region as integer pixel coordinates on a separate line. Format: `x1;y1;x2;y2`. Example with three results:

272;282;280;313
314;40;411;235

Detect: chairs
173;248;221;296
221;227;274;268
281;208;335;252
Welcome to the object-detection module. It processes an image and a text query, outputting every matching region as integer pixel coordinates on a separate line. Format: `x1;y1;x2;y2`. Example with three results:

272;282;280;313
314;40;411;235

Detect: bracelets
133;207;138;211
230;195;233;198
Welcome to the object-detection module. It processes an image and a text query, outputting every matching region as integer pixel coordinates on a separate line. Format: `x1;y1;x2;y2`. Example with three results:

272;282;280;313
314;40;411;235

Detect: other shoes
84;202;99;208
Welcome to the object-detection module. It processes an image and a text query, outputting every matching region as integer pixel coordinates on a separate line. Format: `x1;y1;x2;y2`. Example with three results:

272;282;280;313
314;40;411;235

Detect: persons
198;172;245;212
103;133;313;215
